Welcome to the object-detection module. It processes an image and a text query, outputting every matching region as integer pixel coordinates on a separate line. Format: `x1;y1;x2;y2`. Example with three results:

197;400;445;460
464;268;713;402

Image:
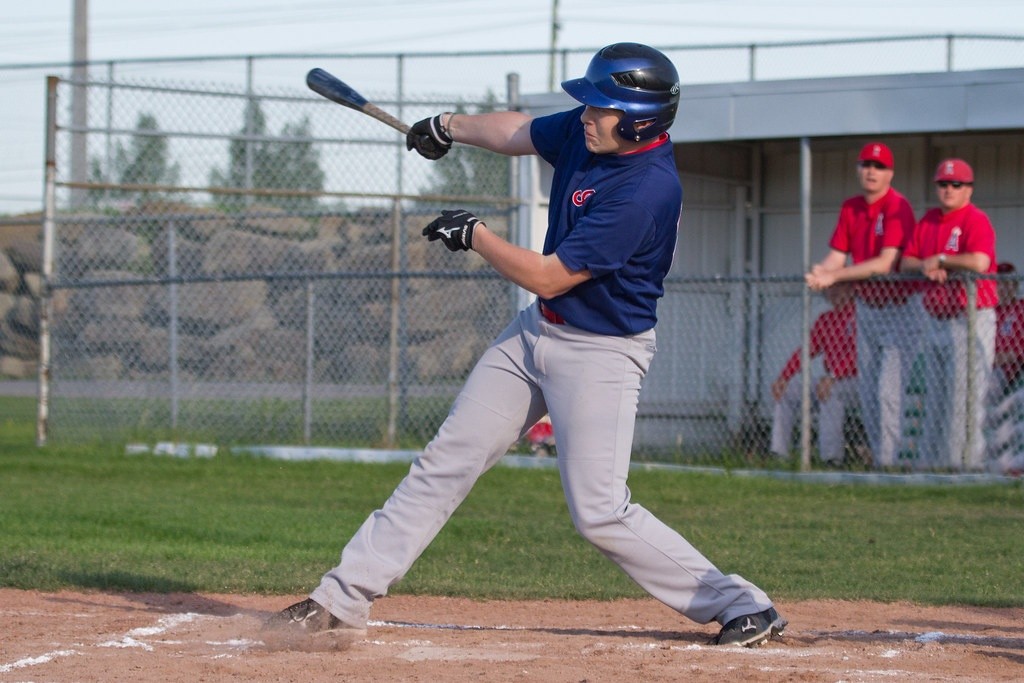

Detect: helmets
561;42;680;142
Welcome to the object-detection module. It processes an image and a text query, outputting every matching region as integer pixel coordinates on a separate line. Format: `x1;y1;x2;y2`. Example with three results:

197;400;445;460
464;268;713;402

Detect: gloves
422;209;486;252
406;114;453;160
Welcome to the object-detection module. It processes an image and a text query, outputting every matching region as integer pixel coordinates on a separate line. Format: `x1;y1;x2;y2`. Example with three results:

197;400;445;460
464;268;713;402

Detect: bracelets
938;253;946;269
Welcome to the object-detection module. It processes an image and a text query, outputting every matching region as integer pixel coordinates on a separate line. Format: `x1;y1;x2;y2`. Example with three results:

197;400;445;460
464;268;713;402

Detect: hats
933;159;974;183
856;142;894;169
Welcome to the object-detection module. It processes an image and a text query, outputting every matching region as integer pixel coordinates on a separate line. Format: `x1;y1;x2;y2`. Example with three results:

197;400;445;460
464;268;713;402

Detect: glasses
939;181;971;187
860;160;886;170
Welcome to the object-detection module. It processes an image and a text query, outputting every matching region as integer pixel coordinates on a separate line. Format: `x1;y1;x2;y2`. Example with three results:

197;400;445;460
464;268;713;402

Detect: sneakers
716;607;788;648
261;599;367;638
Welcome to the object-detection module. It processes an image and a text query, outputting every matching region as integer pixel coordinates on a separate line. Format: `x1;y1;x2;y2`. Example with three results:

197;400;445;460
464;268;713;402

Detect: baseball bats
307;67;436;147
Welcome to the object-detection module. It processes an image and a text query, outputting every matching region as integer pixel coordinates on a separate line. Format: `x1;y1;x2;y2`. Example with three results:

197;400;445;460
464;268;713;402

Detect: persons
804;139;922;472
259;40;787;653
898;158;1002;474
988;262;1024;468
768;284;859;465
